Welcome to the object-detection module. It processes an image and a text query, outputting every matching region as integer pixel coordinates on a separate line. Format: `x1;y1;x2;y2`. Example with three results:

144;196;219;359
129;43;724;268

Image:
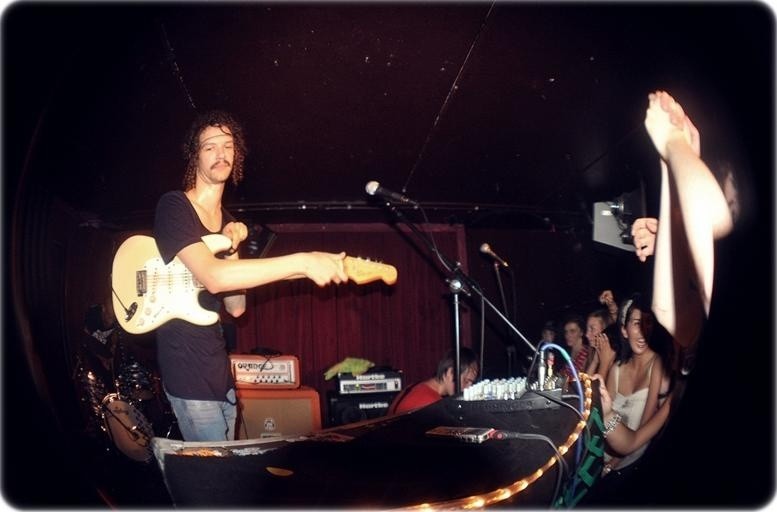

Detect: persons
626;212;656;259
589;321;644;380
385;347;482;418
588;372;686;455
146;107;347;442
558;316;590;384
598;288;620;323
629;88;740;331
533;319;564;380
584;309;608;376
597;298;668;472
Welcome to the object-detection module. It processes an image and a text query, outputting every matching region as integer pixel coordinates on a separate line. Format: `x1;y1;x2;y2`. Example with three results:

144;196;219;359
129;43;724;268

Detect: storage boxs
234;388;321;442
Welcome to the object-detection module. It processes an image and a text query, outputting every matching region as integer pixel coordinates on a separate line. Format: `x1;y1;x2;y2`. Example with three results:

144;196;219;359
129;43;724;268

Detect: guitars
111;235;398;335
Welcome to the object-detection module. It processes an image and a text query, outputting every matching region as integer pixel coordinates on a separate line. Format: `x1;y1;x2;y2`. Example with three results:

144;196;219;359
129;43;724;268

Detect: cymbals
69;326;113;360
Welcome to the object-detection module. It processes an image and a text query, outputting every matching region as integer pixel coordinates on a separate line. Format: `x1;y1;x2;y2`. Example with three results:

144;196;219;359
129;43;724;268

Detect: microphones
478;241;507;269
365;180;416;211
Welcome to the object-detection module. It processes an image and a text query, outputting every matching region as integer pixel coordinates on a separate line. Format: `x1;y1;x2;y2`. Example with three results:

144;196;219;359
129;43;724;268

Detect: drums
117;364;160;400
102;393;154;462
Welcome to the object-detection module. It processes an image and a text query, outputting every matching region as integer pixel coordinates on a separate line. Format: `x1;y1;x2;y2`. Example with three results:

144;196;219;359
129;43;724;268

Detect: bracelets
604;415;622;435
